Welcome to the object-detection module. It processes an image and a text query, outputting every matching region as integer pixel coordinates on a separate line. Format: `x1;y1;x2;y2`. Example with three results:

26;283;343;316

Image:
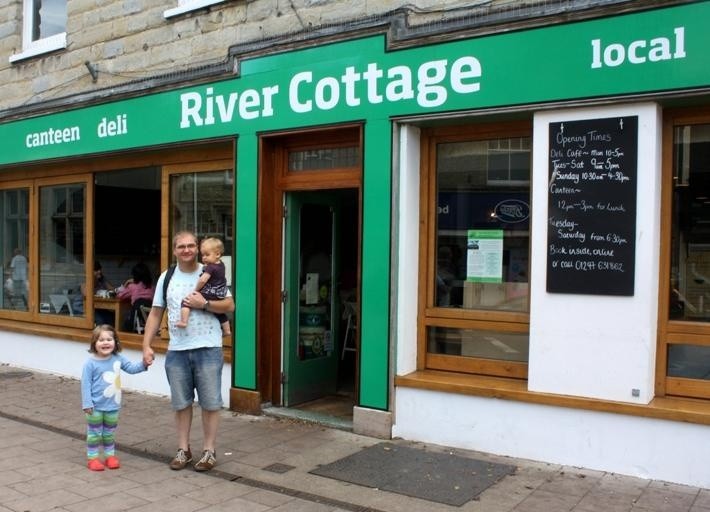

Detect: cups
96;289;116;298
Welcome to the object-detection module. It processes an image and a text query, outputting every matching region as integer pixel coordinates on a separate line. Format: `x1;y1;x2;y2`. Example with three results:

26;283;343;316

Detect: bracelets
203;300;209;312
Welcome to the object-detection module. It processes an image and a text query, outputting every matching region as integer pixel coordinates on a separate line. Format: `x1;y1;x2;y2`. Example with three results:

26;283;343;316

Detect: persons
80;323;152;472
144;230;235;473
79;259;115;329
9;248;30;299
114;262;154;335
436;272;451;354
173;235;235;337
436;245;455;284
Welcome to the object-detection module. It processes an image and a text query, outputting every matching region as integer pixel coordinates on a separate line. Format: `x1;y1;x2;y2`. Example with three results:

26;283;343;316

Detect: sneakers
195;449;217;470
88;458;104;472
105;456;120;469
169;444;193;470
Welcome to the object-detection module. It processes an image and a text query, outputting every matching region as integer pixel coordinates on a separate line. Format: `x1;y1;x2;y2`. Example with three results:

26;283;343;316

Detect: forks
300;306;327;326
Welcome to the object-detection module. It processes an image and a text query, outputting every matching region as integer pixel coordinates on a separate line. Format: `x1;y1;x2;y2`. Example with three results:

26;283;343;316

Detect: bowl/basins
297;326;327;346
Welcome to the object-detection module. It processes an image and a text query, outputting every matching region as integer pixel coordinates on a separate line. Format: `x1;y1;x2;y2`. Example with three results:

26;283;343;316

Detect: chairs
129;298;151;334
65;293;84;316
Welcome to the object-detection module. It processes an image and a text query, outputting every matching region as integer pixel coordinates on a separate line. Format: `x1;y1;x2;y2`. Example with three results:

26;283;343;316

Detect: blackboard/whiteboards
547;117;637;297
